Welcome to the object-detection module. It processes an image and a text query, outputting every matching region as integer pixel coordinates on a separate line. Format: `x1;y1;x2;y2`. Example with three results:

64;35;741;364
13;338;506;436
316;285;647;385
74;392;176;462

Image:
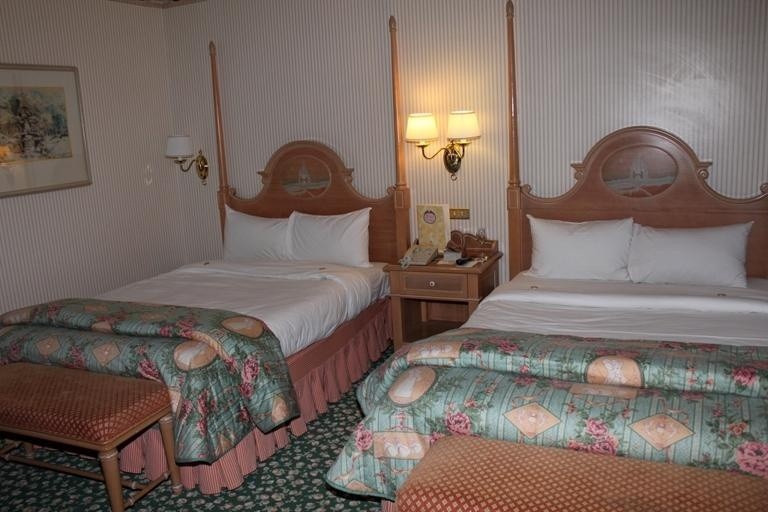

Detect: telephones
403;245;438;265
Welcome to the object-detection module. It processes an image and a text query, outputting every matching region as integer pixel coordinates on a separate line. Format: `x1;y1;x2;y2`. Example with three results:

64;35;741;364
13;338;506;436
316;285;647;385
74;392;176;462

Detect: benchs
0;361;183;512
393;434;767;512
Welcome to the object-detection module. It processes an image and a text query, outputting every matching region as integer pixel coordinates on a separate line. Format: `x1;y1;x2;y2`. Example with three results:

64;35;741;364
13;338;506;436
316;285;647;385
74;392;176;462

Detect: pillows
626;219;755;290
286;207;373;268
523;213;631;283
221;202;289;266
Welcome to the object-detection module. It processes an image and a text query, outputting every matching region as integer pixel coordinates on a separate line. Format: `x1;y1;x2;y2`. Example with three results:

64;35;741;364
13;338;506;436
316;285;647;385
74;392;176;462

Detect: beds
0;13;410;497
320;0;768;512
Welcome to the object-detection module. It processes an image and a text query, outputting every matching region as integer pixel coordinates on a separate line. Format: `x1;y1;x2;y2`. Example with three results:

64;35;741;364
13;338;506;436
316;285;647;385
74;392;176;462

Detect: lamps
163;133;209;188
402;110;483;181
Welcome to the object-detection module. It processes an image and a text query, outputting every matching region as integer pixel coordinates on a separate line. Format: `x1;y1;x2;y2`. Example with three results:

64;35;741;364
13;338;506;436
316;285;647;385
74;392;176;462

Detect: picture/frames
0;61;93;200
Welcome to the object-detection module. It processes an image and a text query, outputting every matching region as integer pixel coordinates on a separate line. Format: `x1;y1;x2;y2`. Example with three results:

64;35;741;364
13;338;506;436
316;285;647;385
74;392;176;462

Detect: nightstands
382;251;503;354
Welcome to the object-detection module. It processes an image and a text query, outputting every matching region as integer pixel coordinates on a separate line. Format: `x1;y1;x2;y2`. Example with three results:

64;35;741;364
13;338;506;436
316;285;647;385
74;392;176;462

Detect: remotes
456;257;472;265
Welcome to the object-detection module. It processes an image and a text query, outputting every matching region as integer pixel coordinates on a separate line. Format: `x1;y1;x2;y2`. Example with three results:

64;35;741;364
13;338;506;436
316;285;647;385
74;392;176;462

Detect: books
416;203;452;252
